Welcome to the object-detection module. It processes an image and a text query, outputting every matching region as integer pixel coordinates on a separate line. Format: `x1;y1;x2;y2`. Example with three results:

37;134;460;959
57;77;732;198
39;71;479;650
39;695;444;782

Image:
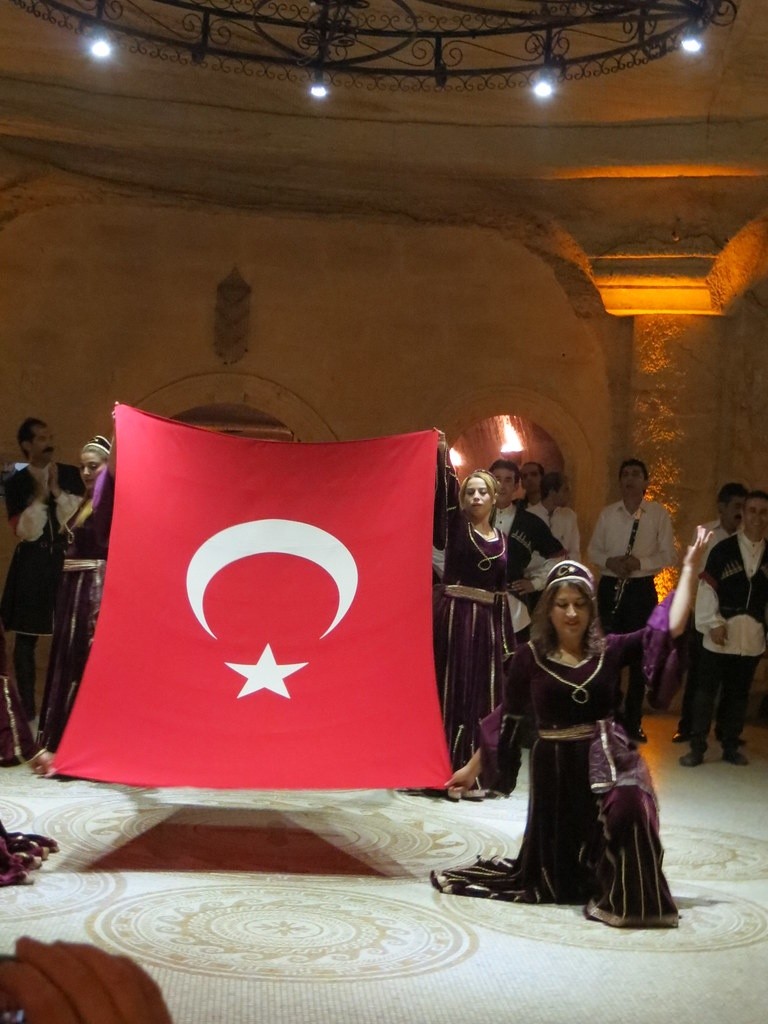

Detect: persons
0;936;171;1024
0;401;118;774
430;426;768;928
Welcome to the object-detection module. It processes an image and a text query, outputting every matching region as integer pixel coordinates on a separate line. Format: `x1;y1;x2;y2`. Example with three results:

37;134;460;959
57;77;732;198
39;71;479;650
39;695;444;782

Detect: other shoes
629;727;646;741
19;704;34;721
721;747;749;764
671;729;693;742
680;748;705;766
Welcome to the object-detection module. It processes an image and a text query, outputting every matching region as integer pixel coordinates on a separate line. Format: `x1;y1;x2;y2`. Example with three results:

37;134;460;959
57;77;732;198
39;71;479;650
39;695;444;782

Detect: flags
55;409;456;794
